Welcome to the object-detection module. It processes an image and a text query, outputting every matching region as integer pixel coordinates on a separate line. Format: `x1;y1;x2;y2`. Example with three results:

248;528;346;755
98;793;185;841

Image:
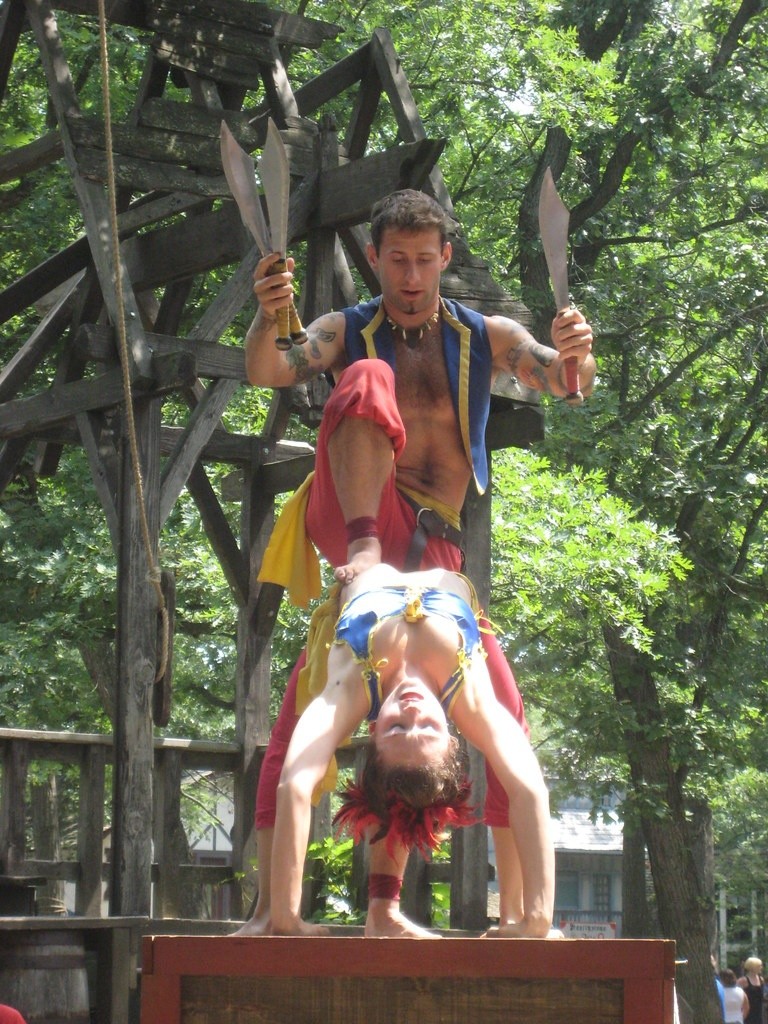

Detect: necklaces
386;313;437;341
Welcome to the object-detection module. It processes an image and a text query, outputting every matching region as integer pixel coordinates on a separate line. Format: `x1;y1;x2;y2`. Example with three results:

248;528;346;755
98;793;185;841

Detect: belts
397;487;468;573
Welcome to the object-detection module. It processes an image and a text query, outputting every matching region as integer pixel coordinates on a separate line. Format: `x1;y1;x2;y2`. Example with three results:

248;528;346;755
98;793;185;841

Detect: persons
711;963;725;1020
246;188;597;938
227;565;557;937
719;969;749;1024
738;957;765;1024
